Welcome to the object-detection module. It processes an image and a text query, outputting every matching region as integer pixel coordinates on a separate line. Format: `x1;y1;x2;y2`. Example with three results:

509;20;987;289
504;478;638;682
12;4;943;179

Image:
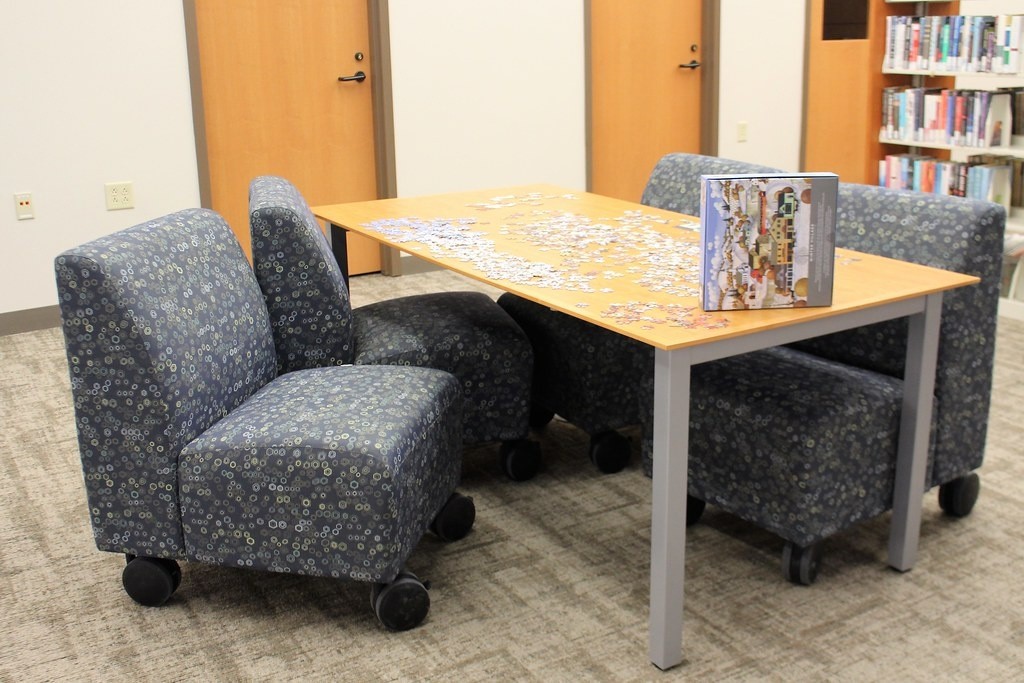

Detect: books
878;11;1022;221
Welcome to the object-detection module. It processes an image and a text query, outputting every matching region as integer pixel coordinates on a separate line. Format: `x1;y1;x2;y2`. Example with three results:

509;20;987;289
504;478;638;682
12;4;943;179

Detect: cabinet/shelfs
863;1;1024;237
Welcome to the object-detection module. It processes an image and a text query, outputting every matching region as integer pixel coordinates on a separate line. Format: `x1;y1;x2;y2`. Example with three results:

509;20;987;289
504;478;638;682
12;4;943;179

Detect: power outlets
106;183;134;211
15;193;35;221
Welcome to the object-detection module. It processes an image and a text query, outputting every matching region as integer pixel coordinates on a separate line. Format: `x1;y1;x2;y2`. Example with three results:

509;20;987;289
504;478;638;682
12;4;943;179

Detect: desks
309;182;982;665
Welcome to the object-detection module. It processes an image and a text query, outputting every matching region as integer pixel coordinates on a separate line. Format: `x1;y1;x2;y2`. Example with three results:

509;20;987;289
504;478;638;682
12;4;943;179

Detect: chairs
643;182;1007;587
53;207;478;635
494;153;786;475
247;175;546;483
